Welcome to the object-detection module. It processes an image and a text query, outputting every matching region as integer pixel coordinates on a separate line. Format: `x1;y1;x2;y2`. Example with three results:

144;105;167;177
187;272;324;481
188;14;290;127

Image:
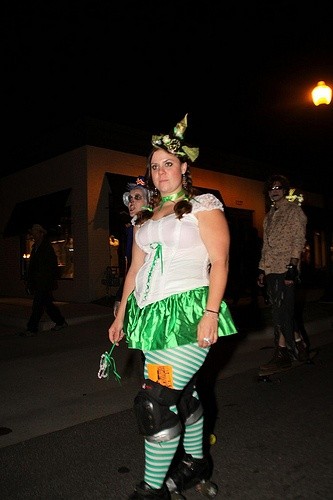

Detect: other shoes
19;328;38;337
274;348;292;368
293;344;309;361
51;321;68;331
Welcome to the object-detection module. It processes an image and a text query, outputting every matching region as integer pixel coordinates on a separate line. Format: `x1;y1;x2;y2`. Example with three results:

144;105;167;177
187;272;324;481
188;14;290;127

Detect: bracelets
204;309;219;321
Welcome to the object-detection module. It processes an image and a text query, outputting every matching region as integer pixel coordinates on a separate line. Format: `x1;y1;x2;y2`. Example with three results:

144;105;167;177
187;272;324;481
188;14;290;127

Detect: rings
203;338;210;343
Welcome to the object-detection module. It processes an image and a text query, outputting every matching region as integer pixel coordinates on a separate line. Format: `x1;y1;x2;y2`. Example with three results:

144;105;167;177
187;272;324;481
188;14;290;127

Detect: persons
256;174;312;370
108;132;240;500
18;223;68;337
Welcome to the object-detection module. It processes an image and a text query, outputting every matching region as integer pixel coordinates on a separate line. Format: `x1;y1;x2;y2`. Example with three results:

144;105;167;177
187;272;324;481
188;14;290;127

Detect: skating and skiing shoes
166;452;218;500
128;481;171;500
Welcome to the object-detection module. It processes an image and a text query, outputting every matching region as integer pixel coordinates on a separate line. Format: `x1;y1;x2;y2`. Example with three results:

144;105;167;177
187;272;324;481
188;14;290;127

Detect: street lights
309;79;333;310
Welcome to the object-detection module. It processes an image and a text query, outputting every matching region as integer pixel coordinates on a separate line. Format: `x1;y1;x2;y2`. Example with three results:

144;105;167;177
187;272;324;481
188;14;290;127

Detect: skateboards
255;350;318;384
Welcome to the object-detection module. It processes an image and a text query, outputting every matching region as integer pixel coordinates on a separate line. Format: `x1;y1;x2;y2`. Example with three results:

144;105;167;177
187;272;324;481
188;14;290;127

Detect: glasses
268;185;284;191
